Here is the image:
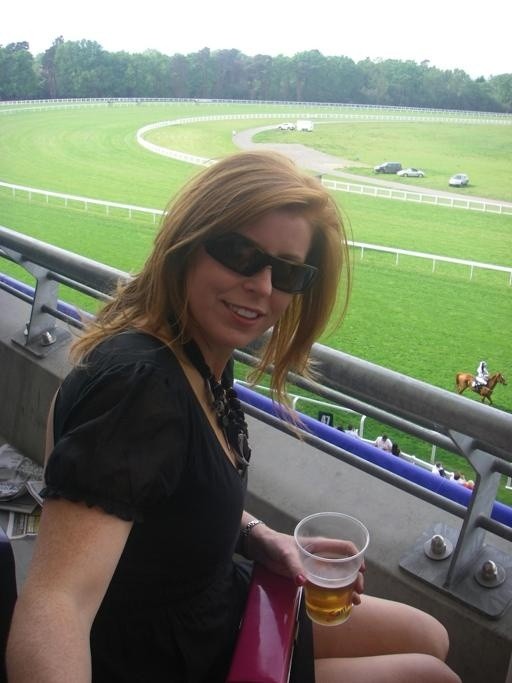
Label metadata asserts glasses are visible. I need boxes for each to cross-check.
[201,223,318,296]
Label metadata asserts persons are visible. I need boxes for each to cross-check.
[431,461,445,477]
[463,479,476,490]
[474,361,489,387]
[374,432,393,451]
[391,442,400,457]
[6,146,466,681]
[447,470,465,484]
[344,423,358,436]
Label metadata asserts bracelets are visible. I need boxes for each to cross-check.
[240,516,265,558]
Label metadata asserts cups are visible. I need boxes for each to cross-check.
[293,512,370,628]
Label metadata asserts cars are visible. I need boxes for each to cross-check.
[278,123,295,130]
[449,174,469,188]
[375,162,424,177]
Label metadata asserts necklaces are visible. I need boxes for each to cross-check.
[167,313,250,465]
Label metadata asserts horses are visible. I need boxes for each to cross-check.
[454,372,508,404]
